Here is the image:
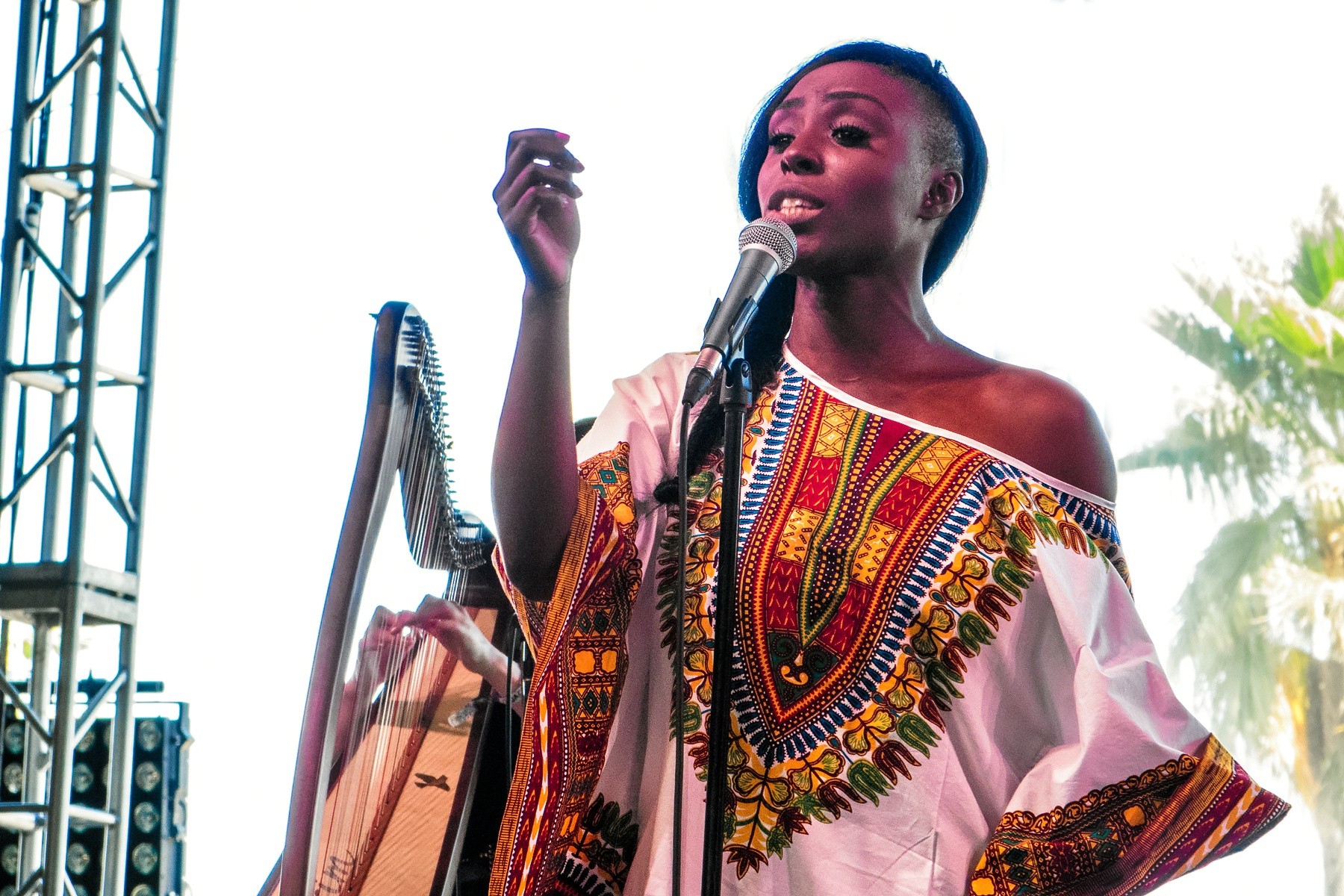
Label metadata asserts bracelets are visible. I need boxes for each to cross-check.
[447,682,523,729]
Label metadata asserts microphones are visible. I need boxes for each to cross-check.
[681,216,797,408]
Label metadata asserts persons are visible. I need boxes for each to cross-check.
[327,40,1292,895]
